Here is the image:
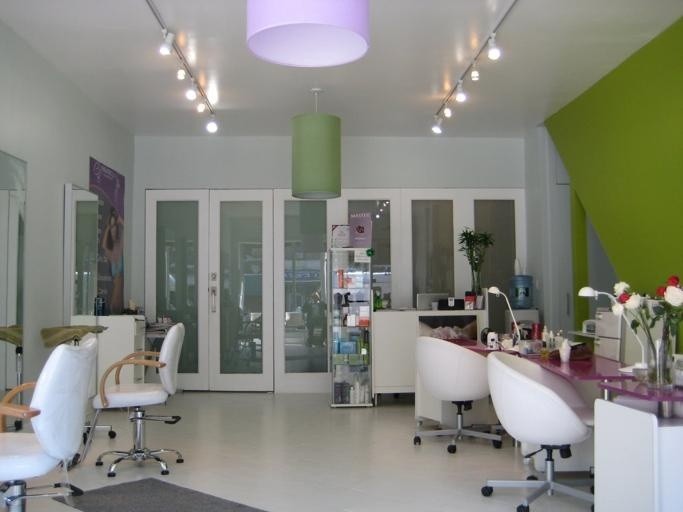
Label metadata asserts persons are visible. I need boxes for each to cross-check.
[102,205,125,315]
[300,289,329,348]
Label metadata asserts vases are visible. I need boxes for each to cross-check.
[649,338,674,390]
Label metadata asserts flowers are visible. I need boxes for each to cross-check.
[610,275,683,392]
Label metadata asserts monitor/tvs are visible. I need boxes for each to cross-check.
[416,293,449,310]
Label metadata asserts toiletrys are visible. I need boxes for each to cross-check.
[333,292,370,327]
[532,323,541,340]
[555,333,564,348]
[333,330,369,365]
[546,330,556,350]
[332,264,370,289]
[559,338,571,363]
[349,381,368,404]
[559,330,565,337]
[334,367,370,404]
[542,325,549,342]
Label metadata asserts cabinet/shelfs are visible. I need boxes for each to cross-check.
[97,315,146,382]
[373,288,491,410]
[326,249,376,412]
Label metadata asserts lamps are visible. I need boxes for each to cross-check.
[577,285,651,375]
[432,117,443,135]
[174,61,186,81]
[289,87,343,202]
[196,101,207,113]
[470,63,481,81]
[454,81,468,102]
[206,117,219,135]
[158,32,174,57]
[486,33,501,60]
[185,84,196,101]
[245,0,371,70]
[442,101,453,120]
[487,282,524,353]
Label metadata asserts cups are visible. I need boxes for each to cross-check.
[432,302,438,310]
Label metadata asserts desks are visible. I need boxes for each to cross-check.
[592,380,682,512]
[424,334,683,475]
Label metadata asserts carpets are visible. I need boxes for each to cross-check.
[54,478,267,512]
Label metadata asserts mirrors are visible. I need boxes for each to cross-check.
[65,182,101,331]
[0,151,28,435]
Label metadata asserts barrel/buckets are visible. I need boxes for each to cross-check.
[508,275,532,309]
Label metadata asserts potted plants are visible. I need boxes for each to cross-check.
[458,227,494,309]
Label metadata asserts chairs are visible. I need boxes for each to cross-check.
[412,333,505,457]
[480,349,592,512]
[1,331,96,512]
[91,322,188,475]
